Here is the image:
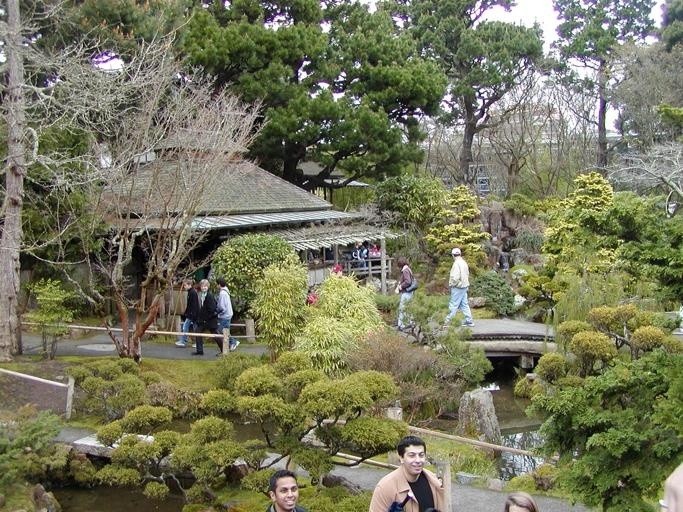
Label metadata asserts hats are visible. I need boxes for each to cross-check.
[452,248,461,255]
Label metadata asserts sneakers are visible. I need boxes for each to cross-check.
[230,341,240,351]
[191,343,196,347]
[462,322,474,326]
[192,351,203,355]
[215,351,222,356]
[175,341,185,347]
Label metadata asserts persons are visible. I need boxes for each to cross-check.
[393,255,417,329]
[350,241,379,281]
[191,278,223,356]
[174,276,200,349]
[503,492,538,512]
[333,259,341,274]
[306,289,314,305]
[308,251,314,263]
[214,279,240,354]
[368,435,450,512]
[441,246,475,327]
[264,469,307,512]
[658,461,682,512]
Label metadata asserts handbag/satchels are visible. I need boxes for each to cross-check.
[404,278,417,292]
